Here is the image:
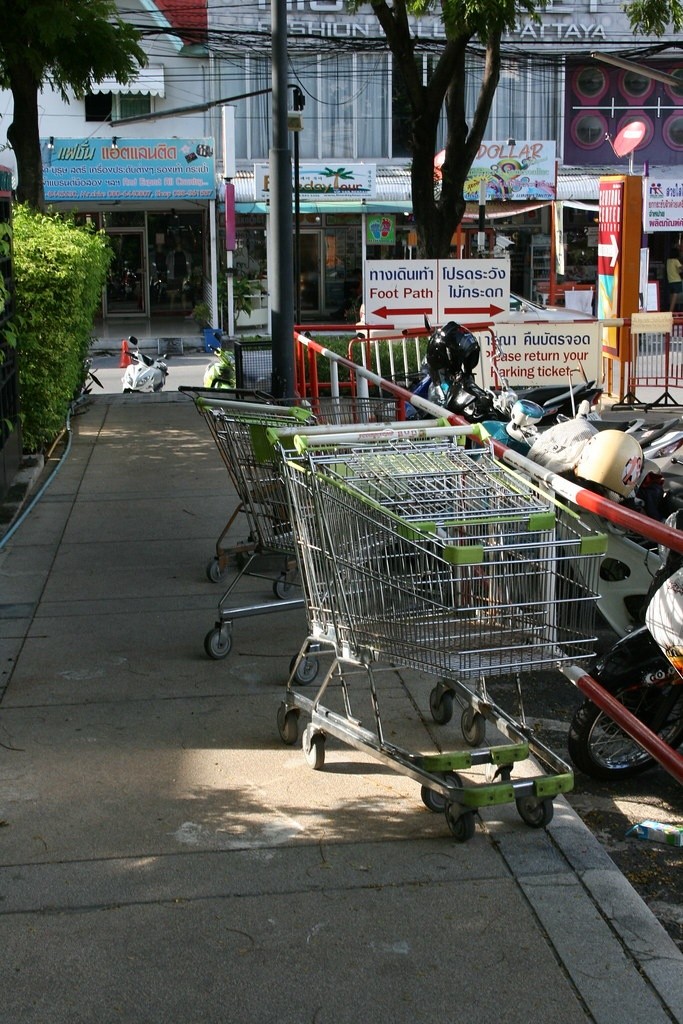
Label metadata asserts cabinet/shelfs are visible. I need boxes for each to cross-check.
[530,233,568,303]
[0,166,25,508]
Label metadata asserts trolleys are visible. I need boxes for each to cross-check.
[292,421,606,841]
[264,415,532,811]
[178,386,305,600]
[192,396,399,688]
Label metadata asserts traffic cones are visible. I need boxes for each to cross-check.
[118,340,134,368]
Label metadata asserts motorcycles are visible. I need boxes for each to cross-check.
[403,318,683,784]
[122,335,169,394]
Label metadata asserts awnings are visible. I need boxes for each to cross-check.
[72,62,165,99]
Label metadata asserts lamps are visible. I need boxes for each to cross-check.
[48,136,54,150]
[111,136,122,149]
[605,119,646,159]
[434,148,446,172]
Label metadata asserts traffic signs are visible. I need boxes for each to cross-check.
[596,181,624,276]
[362,259,438,331]
[437,258,511,325]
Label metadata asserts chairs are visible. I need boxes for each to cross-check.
[535,281,596,308]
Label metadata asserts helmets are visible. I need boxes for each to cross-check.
[576,430,644,498]
[426,319,479,373]
